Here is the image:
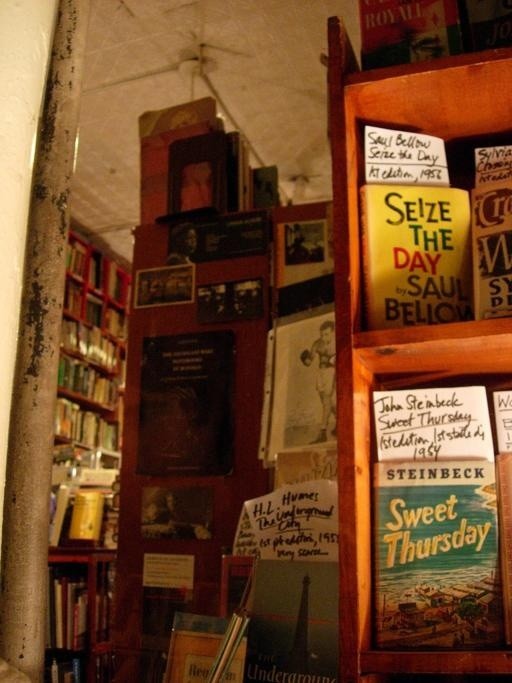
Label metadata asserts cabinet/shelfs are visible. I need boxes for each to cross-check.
[115,200,340,682]
[326,15,512,683]
[44,219,133,681]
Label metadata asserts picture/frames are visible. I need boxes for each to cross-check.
[134,264,196,308]
[195,276,268,324]
[247,558,339,682]
[258,303,337,466]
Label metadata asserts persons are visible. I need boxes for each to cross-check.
[162,221,200,266]
[298,321,338,443]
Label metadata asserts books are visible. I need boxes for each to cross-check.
[108,261,131,345]
[45,401,118,449]
[371,386,512,653]
[359,125,510,329]
[52,354,118,410]
[39,445,123,550]
[57,318,117,366]
[41,564,118,682]
[167,130,297,215]
[60,239,106,328]
